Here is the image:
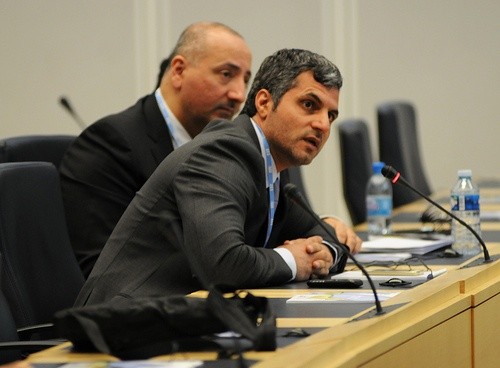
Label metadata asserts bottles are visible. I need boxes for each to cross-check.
[451,170,482,255]
[366,162,393,240]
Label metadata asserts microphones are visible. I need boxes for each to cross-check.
[60,98,86,130]
[381,166,490,261]
[284,183,382,313]
[160,212,247,368]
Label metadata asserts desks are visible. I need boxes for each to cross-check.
[0,186,500,368]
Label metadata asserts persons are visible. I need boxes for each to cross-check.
[60,21,363,283]
[72,48,349,310]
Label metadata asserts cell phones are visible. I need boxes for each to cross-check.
[307,280,363,289]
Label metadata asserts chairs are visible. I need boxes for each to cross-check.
[0,161,90,359]
[377,100,431,209]
[338,119,375,228]
